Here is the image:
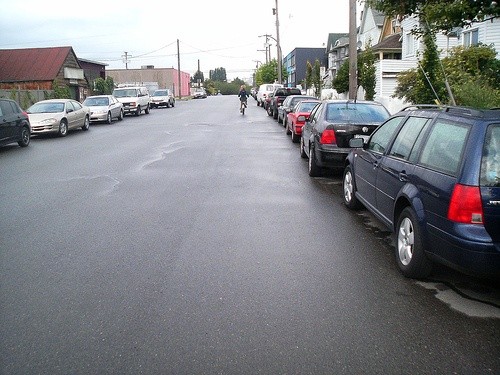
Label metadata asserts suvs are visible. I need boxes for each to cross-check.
[341,104,500,297]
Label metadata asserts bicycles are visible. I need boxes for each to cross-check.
[238,95,249,115]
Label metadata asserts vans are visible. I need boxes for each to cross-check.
[250,83,302,119]
[112,86,151,117]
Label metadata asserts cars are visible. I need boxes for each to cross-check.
[296,100,395,177]
[284,100,322,143]
[0,98,31,149]
[150,89,175,108]
[277,95,319,129]
[193,89,207,99]
[25,98,90,137]
[82,95,124,124]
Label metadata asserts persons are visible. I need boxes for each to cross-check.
[238,85,249,112]
